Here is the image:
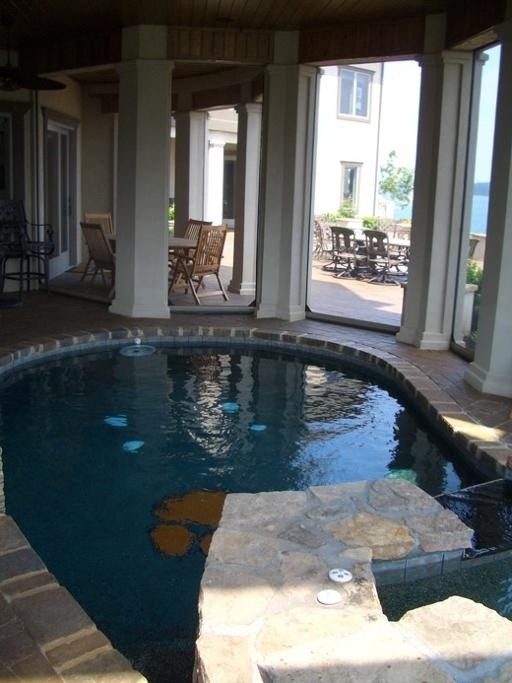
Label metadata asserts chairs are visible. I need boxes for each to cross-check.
[314,216,481,287]
[80,210,229,306]
[0,199,55,307]
